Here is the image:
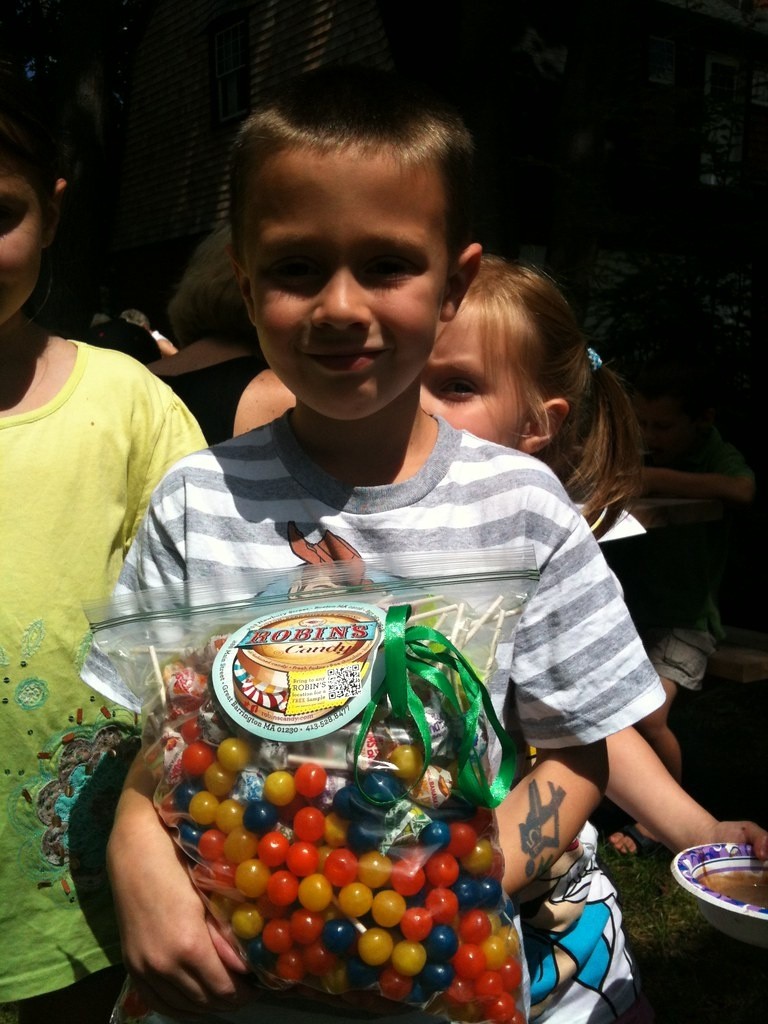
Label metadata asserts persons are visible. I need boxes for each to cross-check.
[77,83,768,1024]
[414,250,658,1022]
[0,70,209,1024]
[146,217,296,449]
[604,349,757,862]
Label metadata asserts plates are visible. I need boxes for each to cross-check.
[671,841,768,951]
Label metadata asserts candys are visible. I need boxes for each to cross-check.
[149,617,528,1024]
